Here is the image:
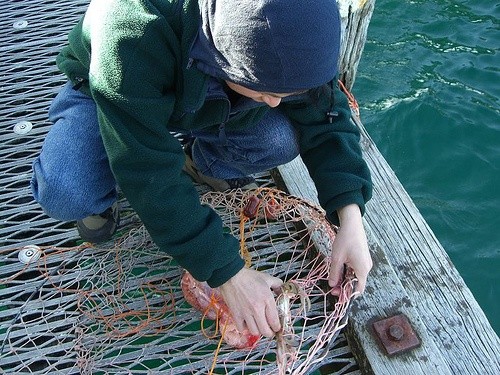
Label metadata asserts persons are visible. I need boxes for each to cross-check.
[31,0,374,338]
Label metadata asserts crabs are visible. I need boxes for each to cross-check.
[275,280,311,347]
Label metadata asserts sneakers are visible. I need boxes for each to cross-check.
[182,137,263,208]
[77,199,119,243]
